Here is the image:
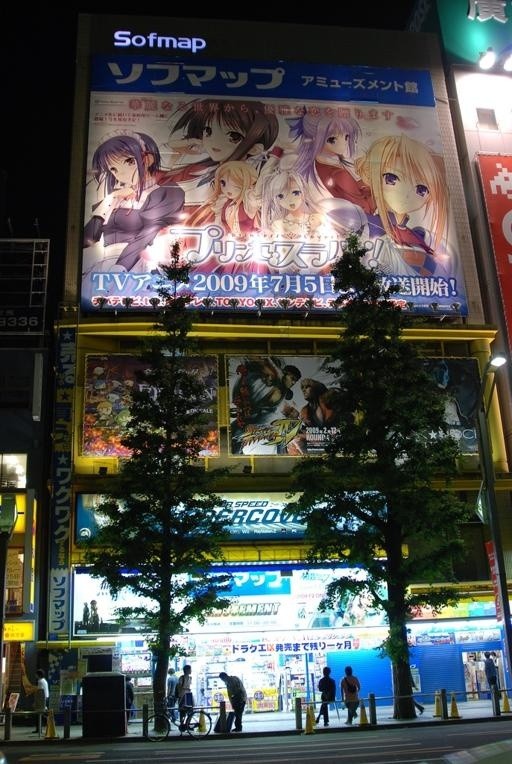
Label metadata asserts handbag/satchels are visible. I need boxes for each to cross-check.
[347,683,357,692]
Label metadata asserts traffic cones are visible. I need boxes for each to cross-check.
[357,697,369,725]
[194,700,209,733]
[431,688,445,718]
[43,706,61,740]
[498,686,512,713]
[307,699,318,727]
[448,691,462,717]
[303,706,316,735]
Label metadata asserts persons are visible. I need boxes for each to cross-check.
[316,667,335,727]
[126,676,133,724]
[167,664,194,725]
[31,669,50,734]
[484,651,501,698]
[341,667,360,725]
[228,354,356,457]
[219,672,247,732]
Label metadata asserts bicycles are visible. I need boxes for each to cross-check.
[141,686,213,742]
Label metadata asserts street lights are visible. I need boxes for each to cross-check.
[467,349,511,691]
